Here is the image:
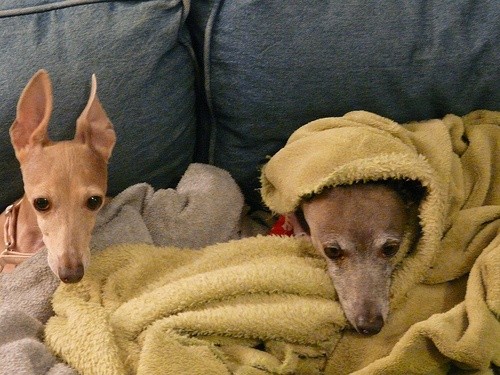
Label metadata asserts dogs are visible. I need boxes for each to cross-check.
[299,184,426,334]
[1,69,116,285]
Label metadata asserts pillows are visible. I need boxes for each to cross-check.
[1,0,202,212]
[187,0,500,213]
